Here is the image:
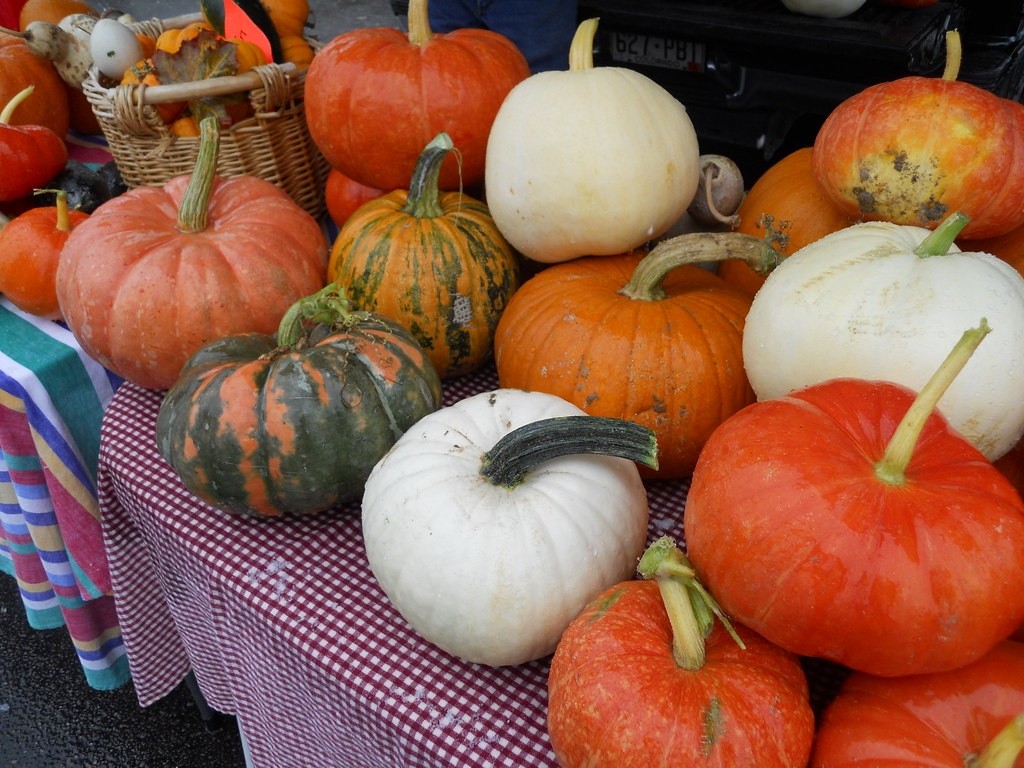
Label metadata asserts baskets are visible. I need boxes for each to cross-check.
[80,9,331,225]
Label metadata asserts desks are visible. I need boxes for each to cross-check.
[0,131,694,768]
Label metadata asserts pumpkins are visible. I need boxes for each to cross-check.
[0,0,1024,768]
